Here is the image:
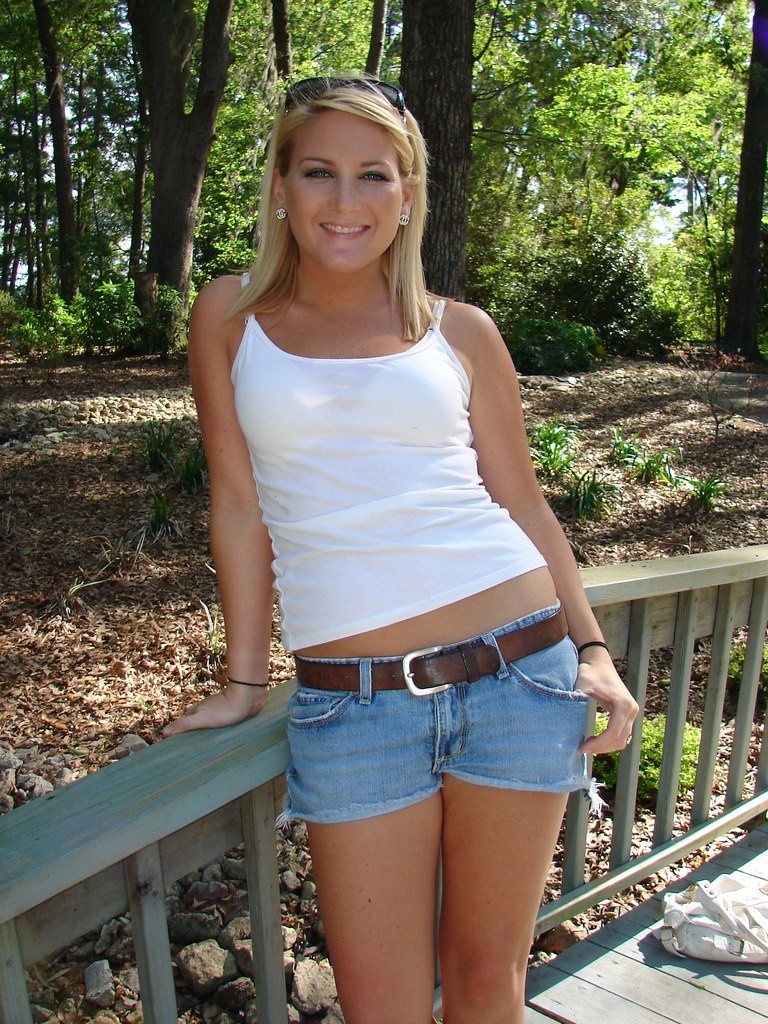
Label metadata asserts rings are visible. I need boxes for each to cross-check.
[627,736,632,744]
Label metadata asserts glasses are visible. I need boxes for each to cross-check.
[283,77,411,142]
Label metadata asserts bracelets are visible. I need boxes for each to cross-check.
[228,677,269,687]
[578,640,609,654]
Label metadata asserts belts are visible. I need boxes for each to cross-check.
[294,602,568,696]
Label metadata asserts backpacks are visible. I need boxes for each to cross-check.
[652,874,768,963]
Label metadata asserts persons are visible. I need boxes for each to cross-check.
[163,76,642,1024]
